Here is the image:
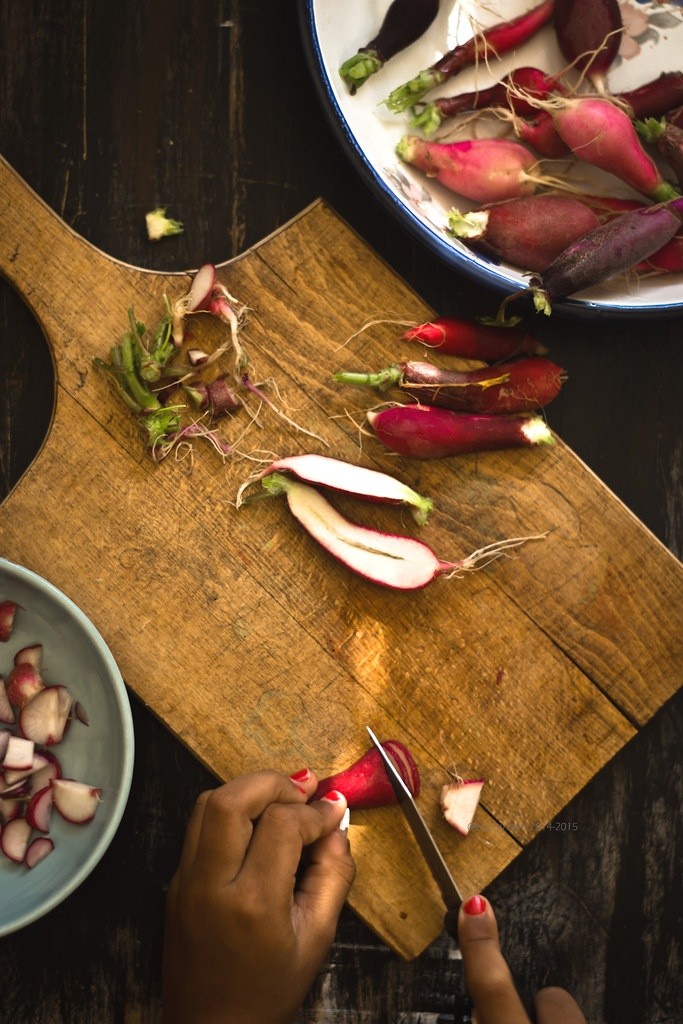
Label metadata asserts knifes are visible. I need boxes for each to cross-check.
[367,726,463,943]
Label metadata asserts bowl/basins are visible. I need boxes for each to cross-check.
[0,559,134,936]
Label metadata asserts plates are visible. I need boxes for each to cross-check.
[312,0,682,313]
[0,265,58,506]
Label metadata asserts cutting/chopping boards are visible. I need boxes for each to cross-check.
[0,158,682,957]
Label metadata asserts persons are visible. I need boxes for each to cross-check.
[159,768,588,1024]
